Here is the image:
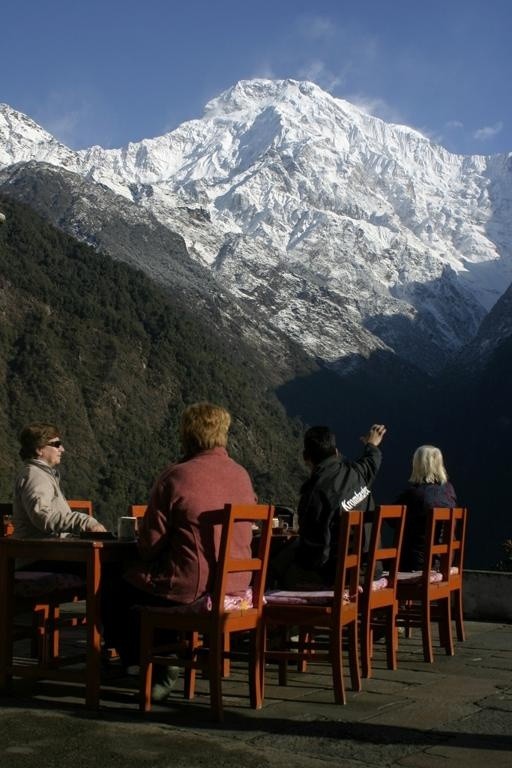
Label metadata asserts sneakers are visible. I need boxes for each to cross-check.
[134,665,178,701]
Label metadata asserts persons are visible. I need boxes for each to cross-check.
[99,402,258,699]
[265,422,386,651]
[374,443,459,640]
[12,425,106,656]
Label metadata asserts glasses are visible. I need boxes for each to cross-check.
[47,439,63,448]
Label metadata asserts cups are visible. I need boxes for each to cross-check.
[111,517,136,538]
[272,518,289,534]
